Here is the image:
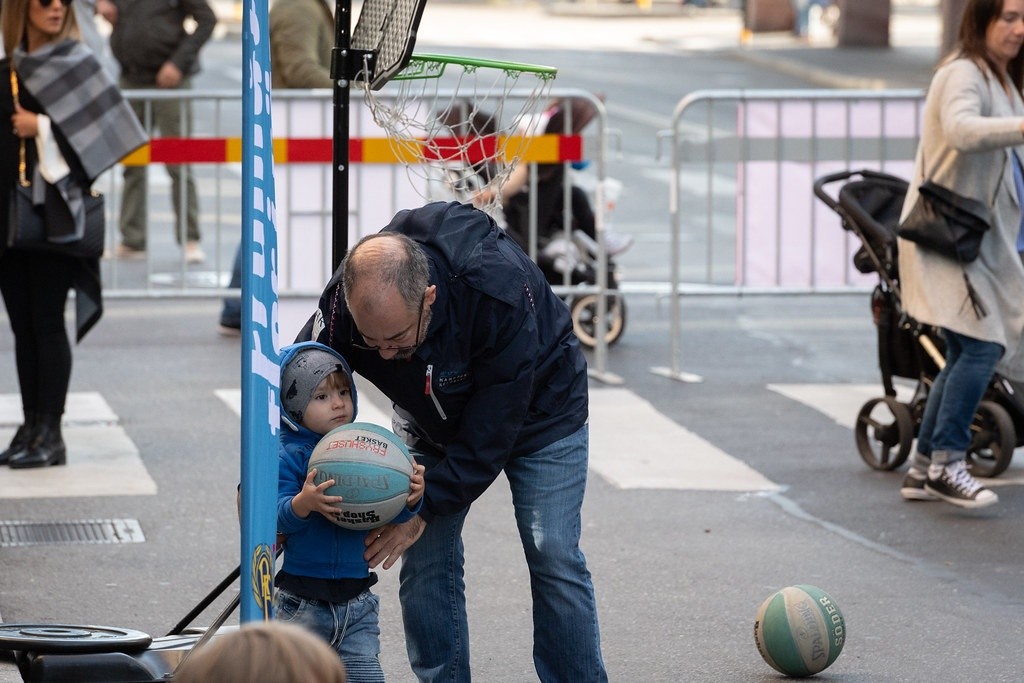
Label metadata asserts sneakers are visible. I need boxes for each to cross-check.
[924,460,999,508]
[901,472,941,501]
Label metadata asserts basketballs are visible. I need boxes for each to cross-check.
[307,422,416,531]
[755,585,846,675]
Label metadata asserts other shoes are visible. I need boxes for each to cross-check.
[102,244,147,261]
[184,240,203,262]
[8,434,66,468]
[0,426,40,465]
[219,302,241,337]
[604,235,632,258]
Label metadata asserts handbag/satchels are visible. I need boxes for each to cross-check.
[897,179,992,321]
[7,183,106,257]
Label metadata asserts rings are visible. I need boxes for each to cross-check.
[13,129,18,134]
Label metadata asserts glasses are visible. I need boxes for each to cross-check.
[39,0,72,8]
[350,287,426,352]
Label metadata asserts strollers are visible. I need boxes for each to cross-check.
[442,90,625,347]
[812,170,1024,479]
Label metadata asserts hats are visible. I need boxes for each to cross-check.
[280,348,350,427]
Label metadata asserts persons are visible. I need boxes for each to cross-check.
[276,339,426,683]
[480,109,599,283]
[98,0,218,264]
[235,198,612,683]
[897,0,1024,510]
[0,0,154,470]
[218,0,369,337]
[173,620,347,683]
